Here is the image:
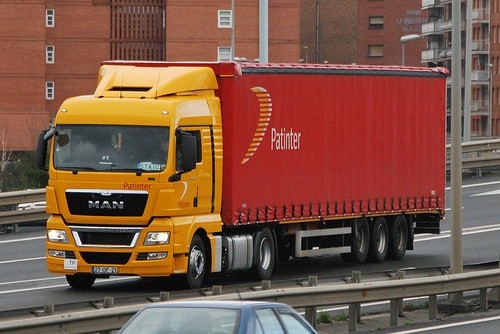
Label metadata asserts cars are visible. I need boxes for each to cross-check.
[117,301,320,334]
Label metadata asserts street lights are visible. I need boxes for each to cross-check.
[398,33,419,67]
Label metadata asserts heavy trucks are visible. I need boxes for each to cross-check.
[36,59,450,290]
